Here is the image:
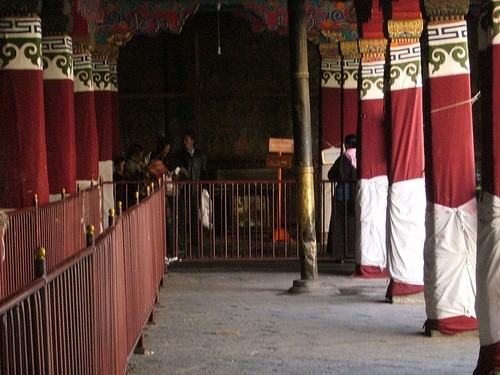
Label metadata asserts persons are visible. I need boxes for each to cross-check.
[114,137,180,258]
[172,131,210,258]
[327,134,357,262]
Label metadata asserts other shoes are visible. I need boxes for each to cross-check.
[189,237,198,247]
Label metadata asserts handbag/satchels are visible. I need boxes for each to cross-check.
[198,188,213,230]
[163,169,183,197]
